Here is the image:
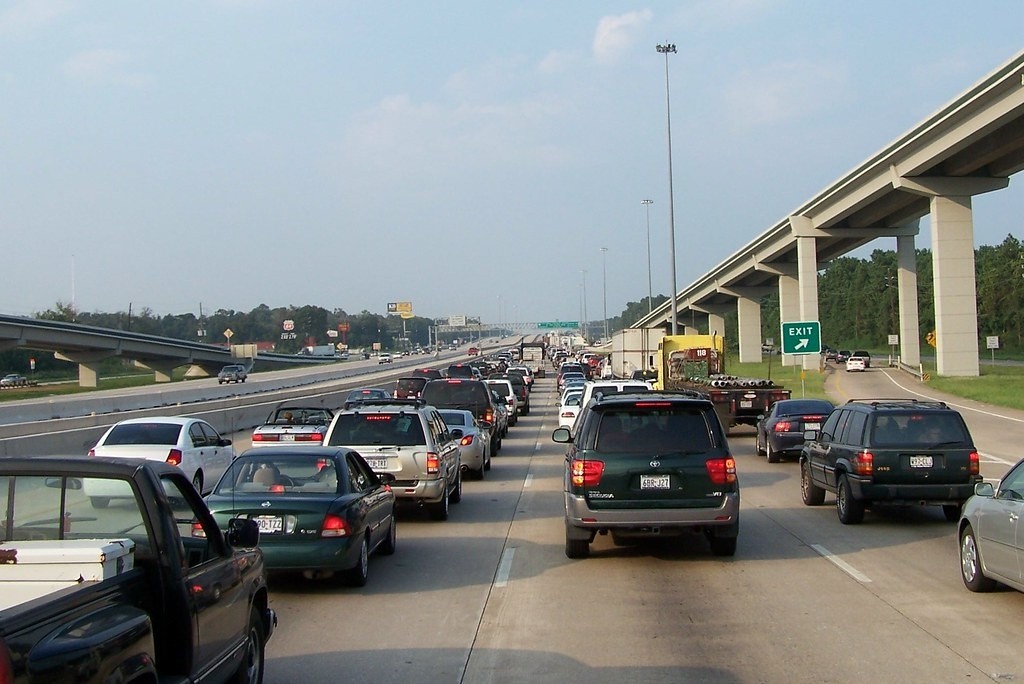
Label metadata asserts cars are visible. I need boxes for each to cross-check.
[0,374,28,388]
[251,331,645,455]
[435,406,495,479]
[83,417,238,510]
[761,343,774,352]
[191,444,398,588]
[821,345,871,372]
[755,399,836,465]
[954,457,1024,595]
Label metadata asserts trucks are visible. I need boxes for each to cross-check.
[632,330,792,437]
[611,327,667,383]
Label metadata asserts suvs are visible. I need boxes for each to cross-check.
[323,397,466,520]
[553,389,740,561]
[217,365,247,385]
[801,397,985,524]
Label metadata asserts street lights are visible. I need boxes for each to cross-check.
[655,40,678,336]
[600,248,609,343]
[580,269,589,339]
[641,199,653,314]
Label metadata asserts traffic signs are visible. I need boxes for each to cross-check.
[782,321,822,356]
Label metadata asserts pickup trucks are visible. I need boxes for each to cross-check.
[0,456,278,684]
